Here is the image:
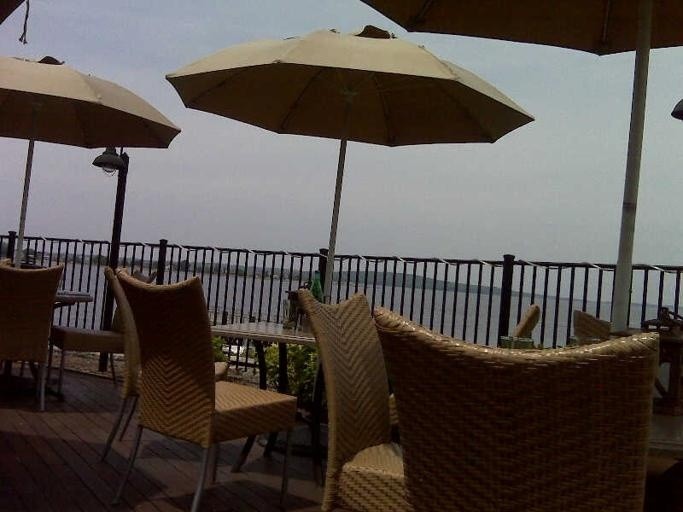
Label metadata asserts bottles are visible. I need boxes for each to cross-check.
[310,270,323,304]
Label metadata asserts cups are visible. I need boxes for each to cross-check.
[295,313,312,337]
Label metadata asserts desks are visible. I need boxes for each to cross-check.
[210,321,327,496]
[19,291,94,395]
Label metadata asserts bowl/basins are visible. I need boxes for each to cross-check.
[500,336,534,349]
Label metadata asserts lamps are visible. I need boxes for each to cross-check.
[90,140,127,176]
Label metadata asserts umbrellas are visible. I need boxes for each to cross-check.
[0,55,181,269]
[360,1,683,341]
[165,24,536,450]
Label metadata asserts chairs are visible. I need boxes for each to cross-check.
[49,272,159,402]
[2,264,62,411]
[374,307,659,512]
[301,289,408,512]
[385,303,683,453]
[100,266,229,459]
[113,271,297,512]
